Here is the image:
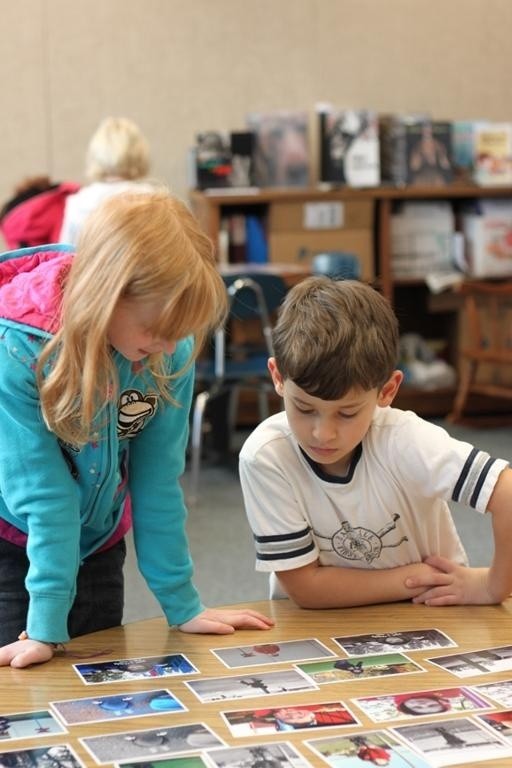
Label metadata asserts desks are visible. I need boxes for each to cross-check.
[0,597,512,768]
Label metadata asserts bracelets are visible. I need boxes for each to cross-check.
[17,630,57,649]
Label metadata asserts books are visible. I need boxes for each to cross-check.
[194,108,512,189]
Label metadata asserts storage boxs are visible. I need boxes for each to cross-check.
[460,199,512,281]
[388,202,455,275]
[262,199,376,285]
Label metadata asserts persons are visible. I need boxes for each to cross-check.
[251,704,324,725]
[237,273,512,610]
[0,188,276,669]
[0,173,82,250]
[334,659,364,674]
[351,736,391,766]
[359,688,463,716]
[435,726,466,751]
[58,116,162,249]
[94,657,170,673]
[346,630,436,645]
[240,677,268,689]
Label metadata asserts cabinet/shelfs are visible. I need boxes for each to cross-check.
[188,183,512,431]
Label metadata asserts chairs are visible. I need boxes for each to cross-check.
[443,278,512,426]
[185,271,289,512]
